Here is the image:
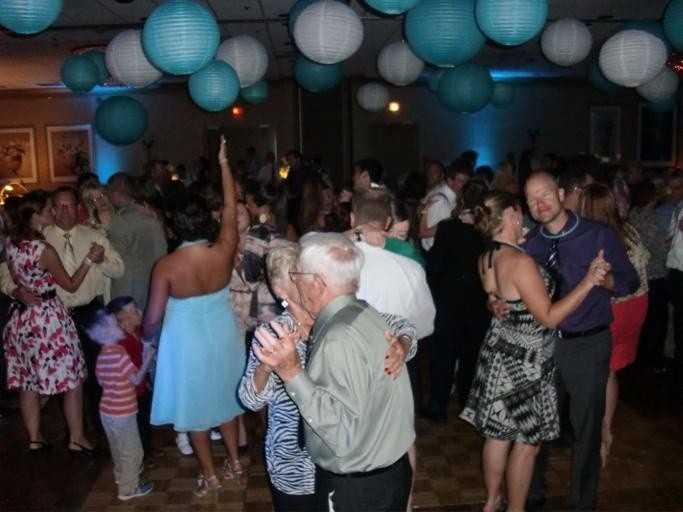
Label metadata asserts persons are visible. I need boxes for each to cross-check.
[1,143,683,512]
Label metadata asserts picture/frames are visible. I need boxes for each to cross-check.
[586,103,623,165]
[44,122,95,184]
[0,126,39,186]
[636,99,678,170]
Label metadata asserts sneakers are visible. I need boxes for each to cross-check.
[114,463,145,485]
[646,364,672,377]
[117,479,154,502]
[209,430,223,441]
[173,433,193,457]
[428,400,448,424]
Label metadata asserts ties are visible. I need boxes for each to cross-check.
[297,324,314,452]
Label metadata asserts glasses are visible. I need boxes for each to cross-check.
[286,269,316,281]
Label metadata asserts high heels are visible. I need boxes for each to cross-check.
[28,440,54,458]
[222,458,247,480]
[192,474,223,500]
[65,439,101,461]
[599,431,614,469]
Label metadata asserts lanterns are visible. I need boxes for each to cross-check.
[59,2,269,143]
[587,1,683,115]
[541,20,592,66]
[288,0,548,116]
[0,0,65,34]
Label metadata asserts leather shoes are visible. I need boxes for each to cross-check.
[238,443,249,456]
